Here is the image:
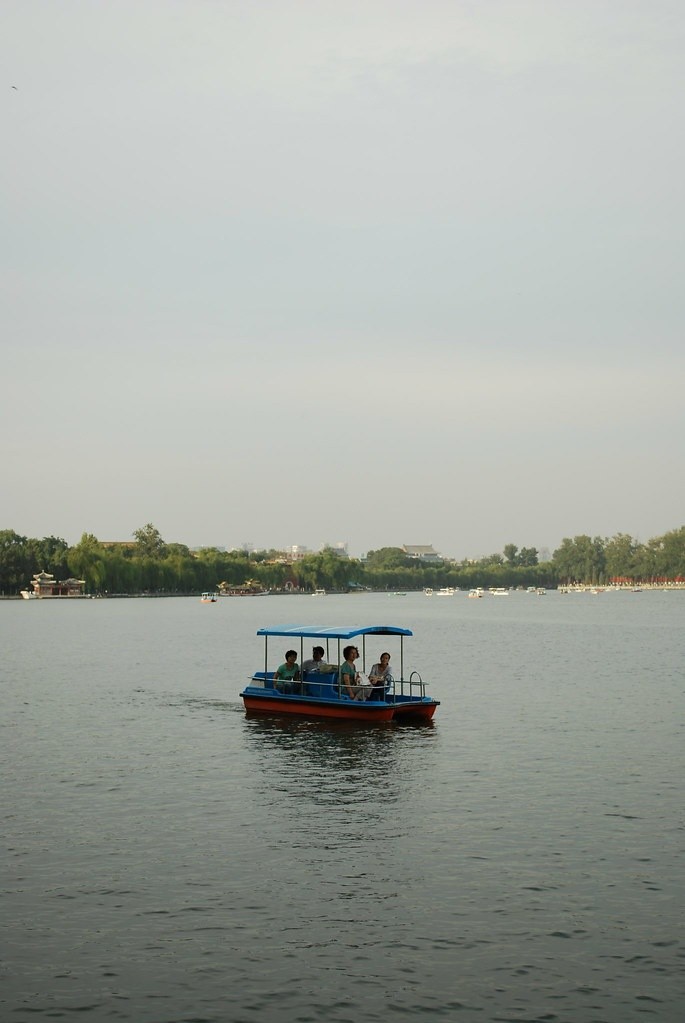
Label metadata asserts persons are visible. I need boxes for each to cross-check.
[300,646,326,672]
[368,652,392,701]
[273,650,300,694]
[341,646,373,702]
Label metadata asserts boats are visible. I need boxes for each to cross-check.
[216,578,271,596]
[312,589,327,597]
[421,585,550,598]
[238,623,441,723]
[199,590,219,603]
